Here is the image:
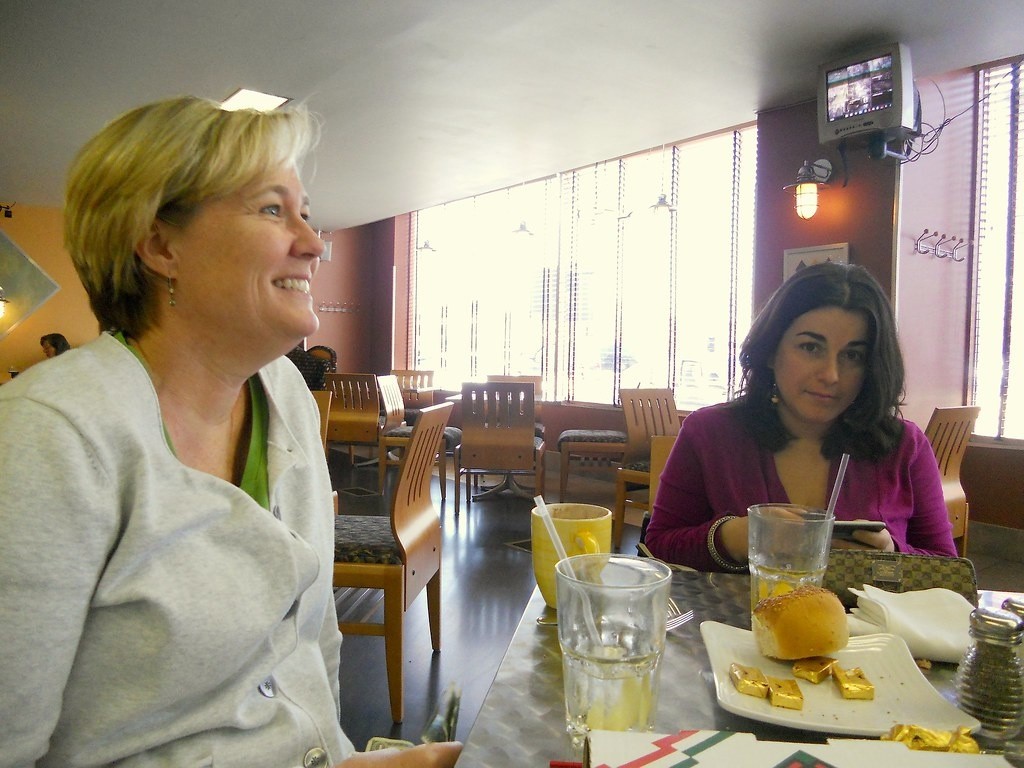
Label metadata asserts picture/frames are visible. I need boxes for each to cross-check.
[782,242,851,284]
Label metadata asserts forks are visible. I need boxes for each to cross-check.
[536,609,693,632]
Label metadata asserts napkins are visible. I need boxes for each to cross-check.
[840,582,985,663]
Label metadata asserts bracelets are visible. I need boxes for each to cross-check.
[706,514,748,573]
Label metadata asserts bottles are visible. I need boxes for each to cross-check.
[954,598,1024,741]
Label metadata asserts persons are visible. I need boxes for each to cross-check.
[644,263,959,577]
[40,333,70,358]
[0,97,465,768]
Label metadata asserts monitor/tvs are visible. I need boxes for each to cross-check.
[817,43,921,152]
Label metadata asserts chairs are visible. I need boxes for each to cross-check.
[279,365,981,724]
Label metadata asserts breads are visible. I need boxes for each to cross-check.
[752,584,850,659]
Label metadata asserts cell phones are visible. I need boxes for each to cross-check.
[831,522,886,547]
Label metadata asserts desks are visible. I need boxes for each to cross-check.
[448,553,1024,767]
[445,391,561,501]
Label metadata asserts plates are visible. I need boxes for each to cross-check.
[700,619,981,734]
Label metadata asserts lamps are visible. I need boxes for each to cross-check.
[0,286,11,317]
[782,158,836,221]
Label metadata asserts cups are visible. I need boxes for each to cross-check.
[555,552,672,743]
[531,502,612,610]
[747,502,836,631]
